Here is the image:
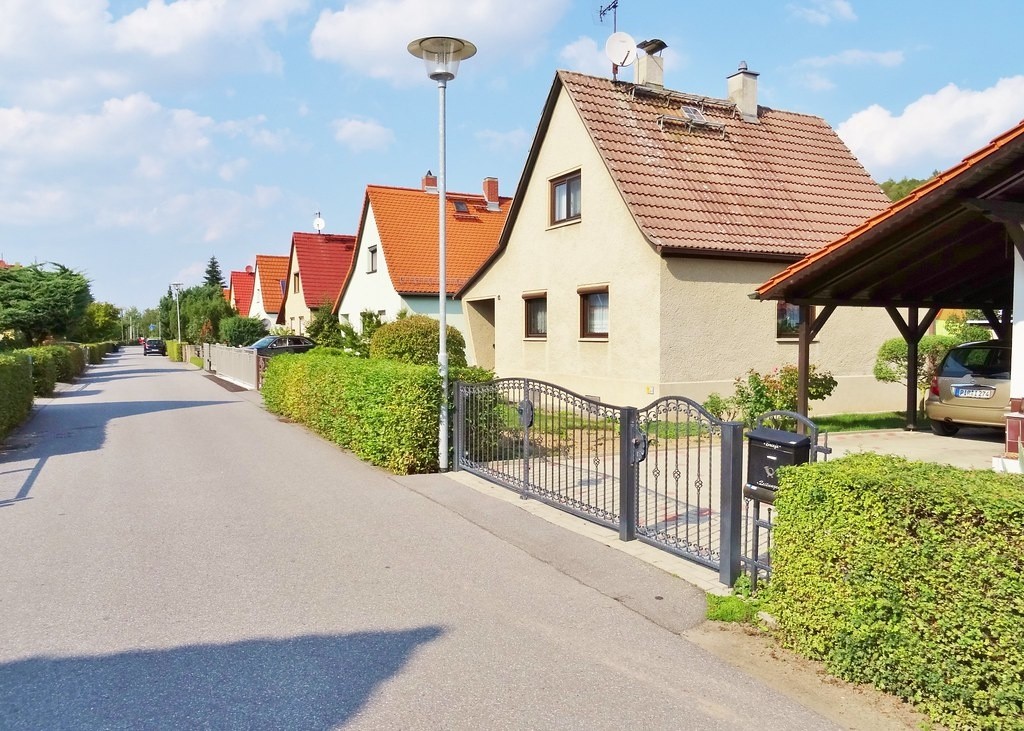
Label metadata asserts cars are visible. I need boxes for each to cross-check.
[244,334,318,358]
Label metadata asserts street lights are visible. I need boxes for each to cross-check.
[170,281,184,343]
[404,34,479,477]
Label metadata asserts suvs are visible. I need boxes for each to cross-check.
[142,339,166,356]
[926,338,1012,436]
